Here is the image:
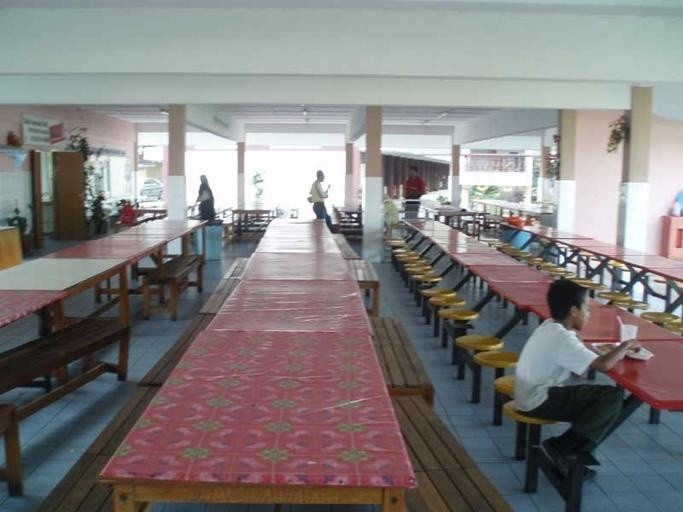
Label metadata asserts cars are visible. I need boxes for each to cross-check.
[136,178,165,202]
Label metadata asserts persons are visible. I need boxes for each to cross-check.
[404,166,426,219]
[193,175,215,221]
[512,277,641,482]
[306,170,334,232]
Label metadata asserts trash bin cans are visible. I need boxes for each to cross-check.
[500,222,531,253]
[197,220,225,260]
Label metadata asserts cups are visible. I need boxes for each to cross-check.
[620,325,637,343]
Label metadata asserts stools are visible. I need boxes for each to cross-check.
[501,399,559,492]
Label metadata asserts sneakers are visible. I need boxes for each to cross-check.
[540,438,561,470]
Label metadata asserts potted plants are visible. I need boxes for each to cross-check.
[85,194,108,235]
[4,197,41,255]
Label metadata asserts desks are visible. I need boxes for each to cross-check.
[1,240,130,381]
